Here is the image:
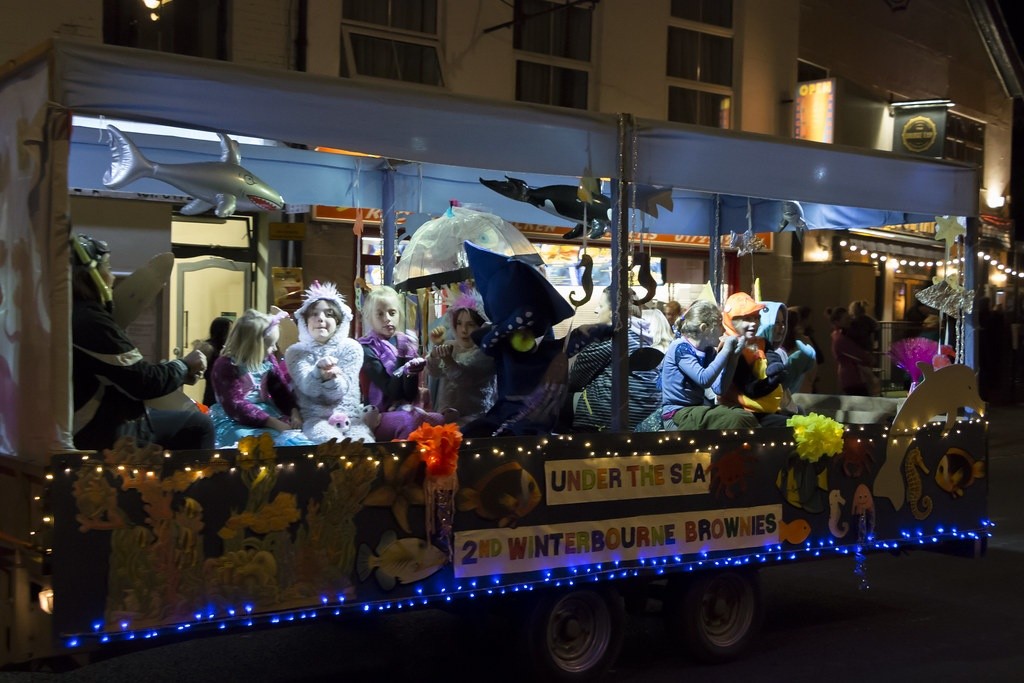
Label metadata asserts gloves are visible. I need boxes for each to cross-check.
[729,334,745,367]
[719,336,738,360]
[785,340,816,380]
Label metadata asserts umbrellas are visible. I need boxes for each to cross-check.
[395,206,543,293]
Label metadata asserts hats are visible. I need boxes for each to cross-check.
[725,292,765,319]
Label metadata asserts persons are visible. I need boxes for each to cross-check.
[285,282,365,443]
[71,235,880,448]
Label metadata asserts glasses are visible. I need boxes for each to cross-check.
[97,256,112,269]
